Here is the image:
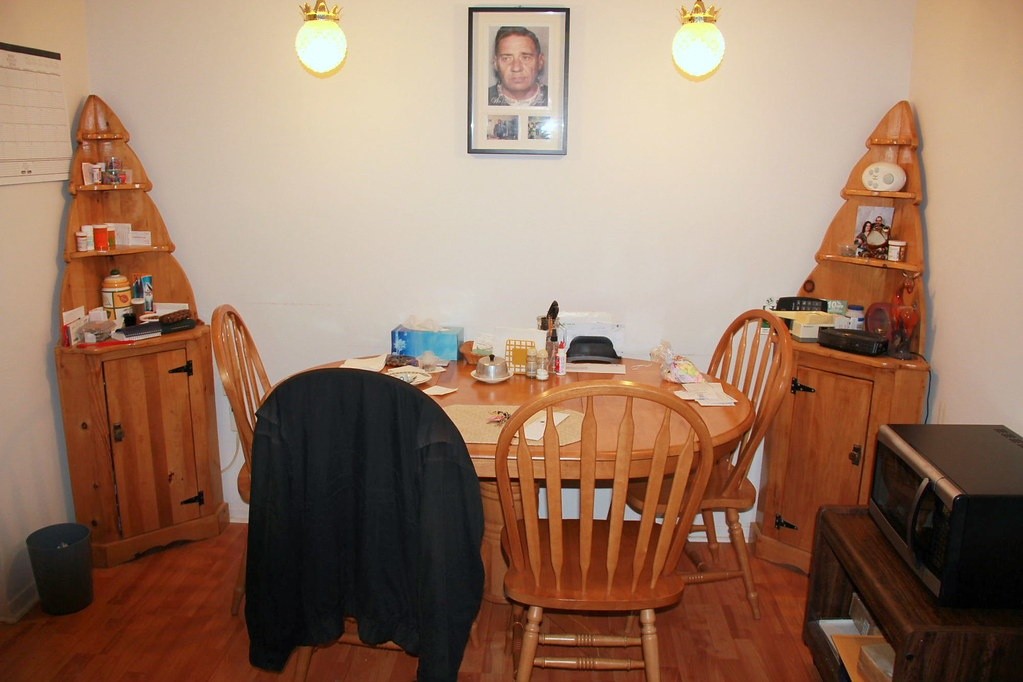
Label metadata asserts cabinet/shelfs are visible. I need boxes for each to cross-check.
[55,95,229,568]
[748,99,931,575]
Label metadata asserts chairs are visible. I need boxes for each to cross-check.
[252,366,483,682]
[607,310,795,619]
[211,305,273,617]
[478,379,713,682]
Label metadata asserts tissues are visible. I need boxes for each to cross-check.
[390,313,464,361]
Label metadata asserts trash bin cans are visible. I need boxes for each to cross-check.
[26,522,94,615]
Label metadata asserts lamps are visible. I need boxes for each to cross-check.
[672,0,726,76]
[294,0,346,74]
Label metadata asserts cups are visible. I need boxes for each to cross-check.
[887,240,908,262]
[130,299,146,322]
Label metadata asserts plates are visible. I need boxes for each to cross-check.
[471,369,514,383]
[385,366,432,386]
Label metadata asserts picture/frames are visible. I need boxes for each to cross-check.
[466,6,570,155]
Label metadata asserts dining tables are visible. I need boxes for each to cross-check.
[257,357,754,606]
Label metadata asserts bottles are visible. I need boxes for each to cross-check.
[526,348,538,379]
[536,349,550,381]
[74,224,116,252]
[845,305,864,330]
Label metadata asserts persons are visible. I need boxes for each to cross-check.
[494,120,505,140]
[488,26,549,107]
[872,216,886,233]
[854,221,871,249]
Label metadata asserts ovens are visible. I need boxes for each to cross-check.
[867,423,1023,605]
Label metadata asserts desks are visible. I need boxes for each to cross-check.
[801,505,1023,682]
